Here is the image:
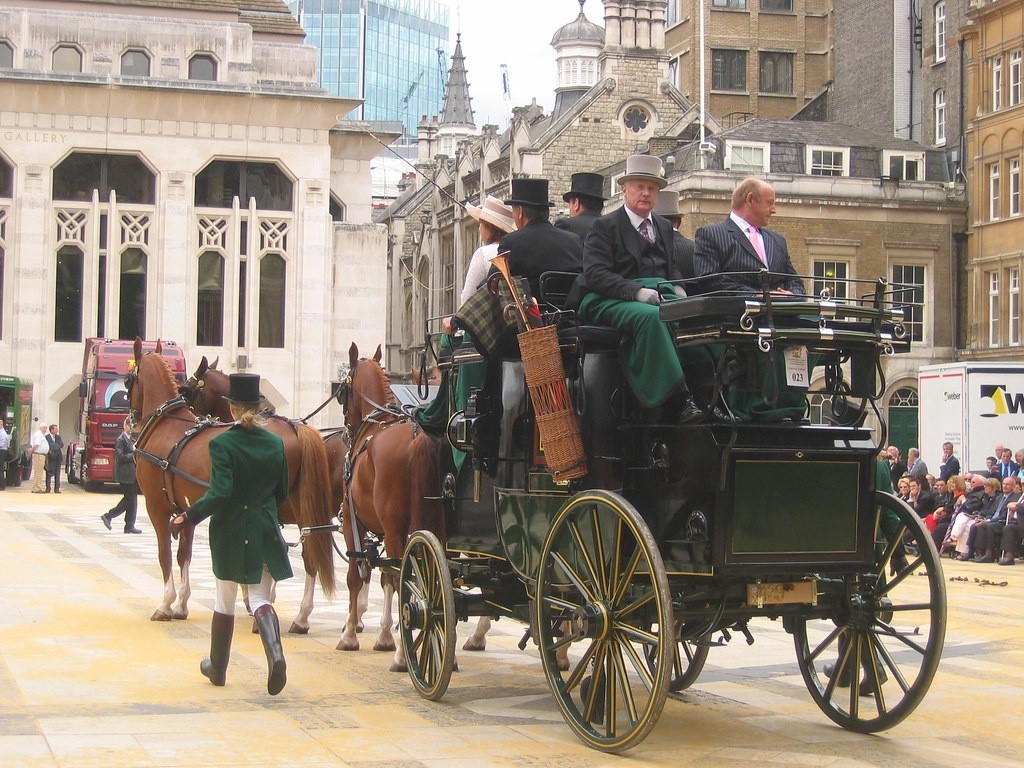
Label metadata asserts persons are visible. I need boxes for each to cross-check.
[877,443,1024,566]
[556,169,610,244]
[461,196,518,308]
[652,190,695,280]
[442,179,585,336]
[45,424,64,494]
[696,179,811,425]
[28,424,49,493]
[100,416,143,534]
[171,373,294,696]
[823,457,913,697]
[567,155,742,424]
[0,420,21,491]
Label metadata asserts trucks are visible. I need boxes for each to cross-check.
[0,375,33,488]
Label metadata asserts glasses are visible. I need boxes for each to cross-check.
[965,478,971,481]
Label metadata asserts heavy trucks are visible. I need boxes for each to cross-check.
[66,338,187,492]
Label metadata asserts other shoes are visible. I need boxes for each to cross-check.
[53,490,61,493]
[45,489,50,493]
[31,489,45,494]
[124,525,143,534]
[791,417,812,426]
[904,545,1016,565]
[100,515,112,530]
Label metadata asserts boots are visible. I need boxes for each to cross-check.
[254,604,287,696]
[855,639,889,698]
[199,611,235,687]
[822,632,853,688]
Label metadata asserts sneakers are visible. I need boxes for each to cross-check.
[704,402,741,425]
[680,398,703,424]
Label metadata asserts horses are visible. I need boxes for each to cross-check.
[129,334,574,673]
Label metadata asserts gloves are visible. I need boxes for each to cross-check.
[636,288,661,306]
[889,556,914,578]
[672,284,688,298]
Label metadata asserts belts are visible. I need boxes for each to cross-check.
[33,452,47,456]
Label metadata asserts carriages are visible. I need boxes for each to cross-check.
[123,268,949,753]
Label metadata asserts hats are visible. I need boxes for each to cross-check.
[651,191,686,219]
[221,373,268,404]
[504,177,557,207]
[464,194,518,233]
[618,154,668,188]
[563,172,609,203]
[898,478,910,488]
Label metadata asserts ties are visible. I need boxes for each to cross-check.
[639,220,649,242]
[748,226,763,264]
[1002,464,1009,479]
[995,496,1006,513]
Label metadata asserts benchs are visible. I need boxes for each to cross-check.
[542,270,835,354]
